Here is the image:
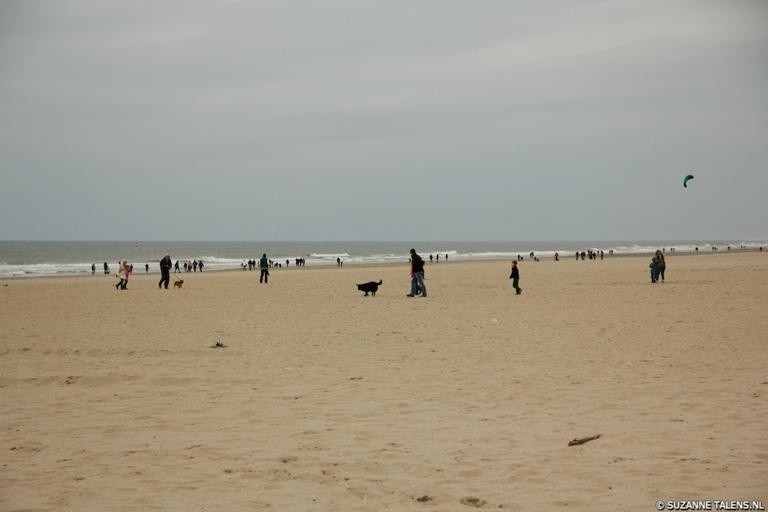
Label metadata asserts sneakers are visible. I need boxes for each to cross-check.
[406,289,427,298]
[114,283,170,290]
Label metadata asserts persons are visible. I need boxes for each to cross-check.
[90,247,666,299]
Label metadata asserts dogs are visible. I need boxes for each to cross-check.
[173,280,184,288]
[356,279,383,296]
